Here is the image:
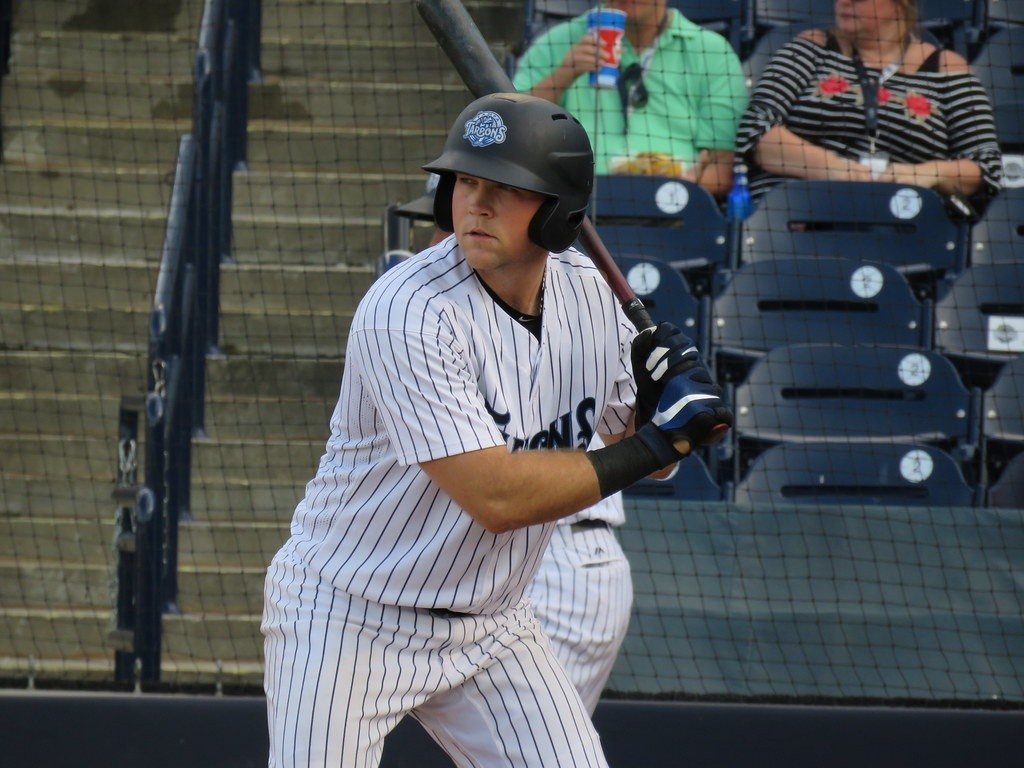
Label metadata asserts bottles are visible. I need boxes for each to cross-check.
[728,166,752,219]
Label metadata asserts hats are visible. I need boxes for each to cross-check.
[394,173,440,221]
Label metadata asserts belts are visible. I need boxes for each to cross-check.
[429,608,468,619]
[571,518,607,528]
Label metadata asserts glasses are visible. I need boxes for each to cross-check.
[621,63,649,108]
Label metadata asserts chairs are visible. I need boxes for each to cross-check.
[381,0,1024,508]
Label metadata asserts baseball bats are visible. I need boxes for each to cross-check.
[415,0,729,448]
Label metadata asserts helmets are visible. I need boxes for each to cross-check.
[422,93,594,254]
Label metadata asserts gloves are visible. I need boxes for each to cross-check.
[633,366,734,469]
[631,322,705,414]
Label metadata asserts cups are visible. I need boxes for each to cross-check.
[587,8,626,87]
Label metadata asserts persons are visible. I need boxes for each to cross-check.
[735,0,1004,316]
[260,93,733,768]
[511,1,748,195]
